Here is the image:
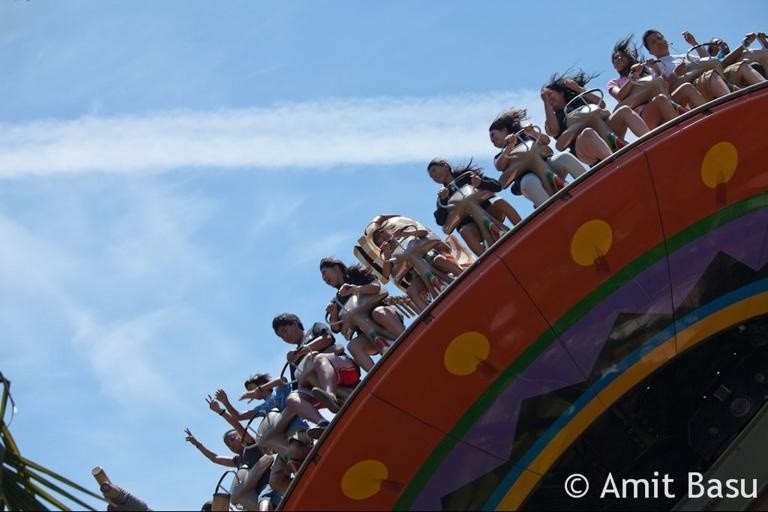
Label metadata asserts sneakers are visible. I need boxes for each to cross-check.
[307,421,329,439]
[312,387,340,413]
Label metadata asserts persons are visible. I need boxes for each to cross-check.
[366,212,464,311]
[184,313,361,512]
[603,26,768,133]
[318,256,409,372]
[488,109,589,210]
[428,154,521,258]
[539,66,651,168]
